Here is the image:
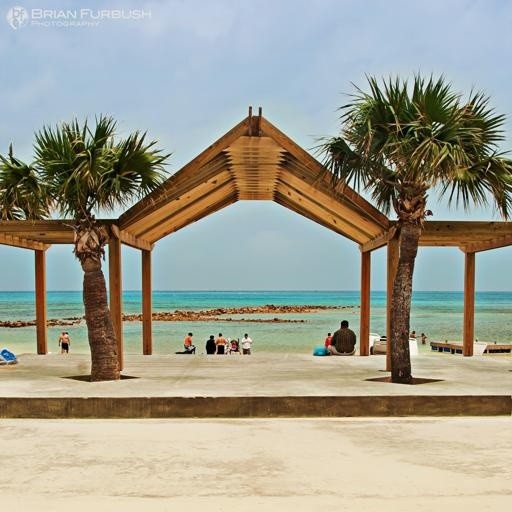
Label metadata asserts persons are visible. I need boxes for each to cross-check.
[410,330,417,338]
[325,331,332,347]
[183,330,253,354]
[420,333,428,345]
[59,331,72,354]
[329,320,357,355]
